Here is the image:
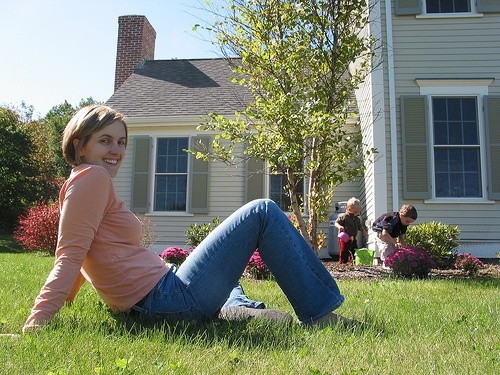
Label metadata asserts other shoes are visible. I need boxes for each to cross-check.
[218,307,293,324]
[299,312,384,335]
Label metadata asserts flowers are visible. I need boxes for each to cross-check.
[453,253,486,273]
[160,246,190,269]
[384,246,437,272]
[245,247,270,278]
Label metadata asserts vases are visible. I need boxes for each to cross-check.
[256,272,271,280]
[393,267,432,281]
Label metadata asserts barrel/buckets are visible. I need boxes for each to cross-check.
[354,249,375,266]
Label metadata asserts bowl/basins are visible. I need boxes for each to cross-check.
[338,231,351,242]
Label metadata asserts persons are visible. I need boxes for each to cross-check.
[371,205,418,270]
[0,104,368,342]
[334,196,368,265]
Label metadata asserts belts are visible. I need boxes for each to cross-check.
[130,298,144,320]
